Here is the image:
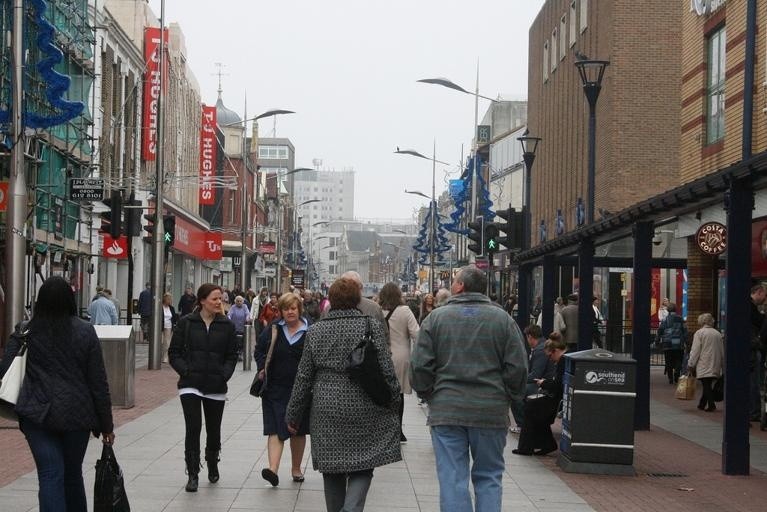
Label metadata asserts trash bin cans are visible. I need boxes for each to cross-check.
[86,324,136,410]
[556,348,638,476]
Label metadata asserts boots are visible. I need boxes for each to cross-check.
[185,450,203,492]
[205,443,221,483]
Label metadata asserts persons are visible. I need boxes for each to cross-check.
[654,303,687,385]
[253,292,315,487]
[504,293,604,456]
[408,266,529,512]
[0,275,116,512]
[167,283,239,492]
[85,286,121,326]
[657,297,669,327]
[748,281,767,427]
[687,313,724,412]
[377,282,421,443]
[284,277,403,511]
[137,270,452,371]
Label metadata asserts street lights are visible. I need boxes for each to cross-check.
[416,66,500,262]
[263,165,332,287]
[575,61,614,349]
[515,131,544,331]
[240,92,295,298]
[386,137,450,295]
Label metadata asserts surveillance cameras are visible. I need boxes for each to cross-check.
[652,234,662,245]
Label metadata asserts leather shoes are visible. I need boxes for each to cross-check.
[292,468,304,482]
[262,469,279,486]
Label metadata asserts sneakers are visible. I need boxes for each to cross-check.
[512,449,532,456]
[535,445,557,455]
[510,426,521,433]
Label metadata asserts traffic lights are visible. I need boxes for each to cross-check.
[143,213,156,246]
[100,193,119,240]
[495,205,516,250]
[164,216,175,245]
[467,216,483,257]
[486,220,500,255]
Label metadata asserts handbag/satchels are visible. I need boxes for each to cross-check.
[250,373,263,397]
[0,342,29,421]
[94,443,131,511]
[713,376,723,402]
[349,317,392,406]
[675,370,698,400]
